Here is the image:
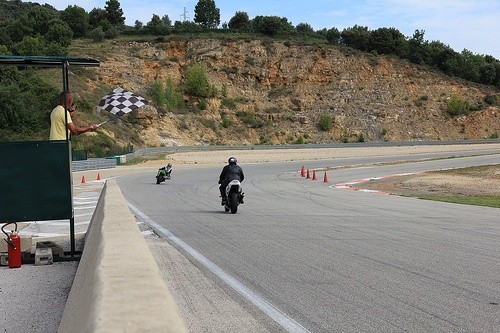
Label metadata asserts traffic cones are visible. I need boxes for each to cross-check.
[323,172,328,183]
[301,166,306,177]
[81,175,85,183]
[312,170,317,180]
[97,173,100,180]
[306,169,310,178]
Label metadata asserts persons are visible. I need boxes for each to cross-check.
[218,158,244,205]
[156,164,172,178]
[49,92,98,140]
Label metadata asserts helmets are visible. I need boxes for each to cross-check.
[228,157,237,163]
[168,163,172,167]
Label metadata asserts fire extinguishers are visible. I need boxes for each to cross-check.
[2,221,21,268]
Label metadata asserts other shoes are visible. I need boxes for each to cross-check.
[240,195,244,204]
[221,195,226,206]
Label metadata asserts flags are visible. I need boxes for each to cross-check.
[97,87,149,117]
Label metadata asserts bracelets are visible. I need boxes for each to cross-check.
[91,126,93,131]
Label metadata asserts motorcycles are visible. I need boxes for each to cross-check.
[156,169,170,184]
[218,180,245,214]
